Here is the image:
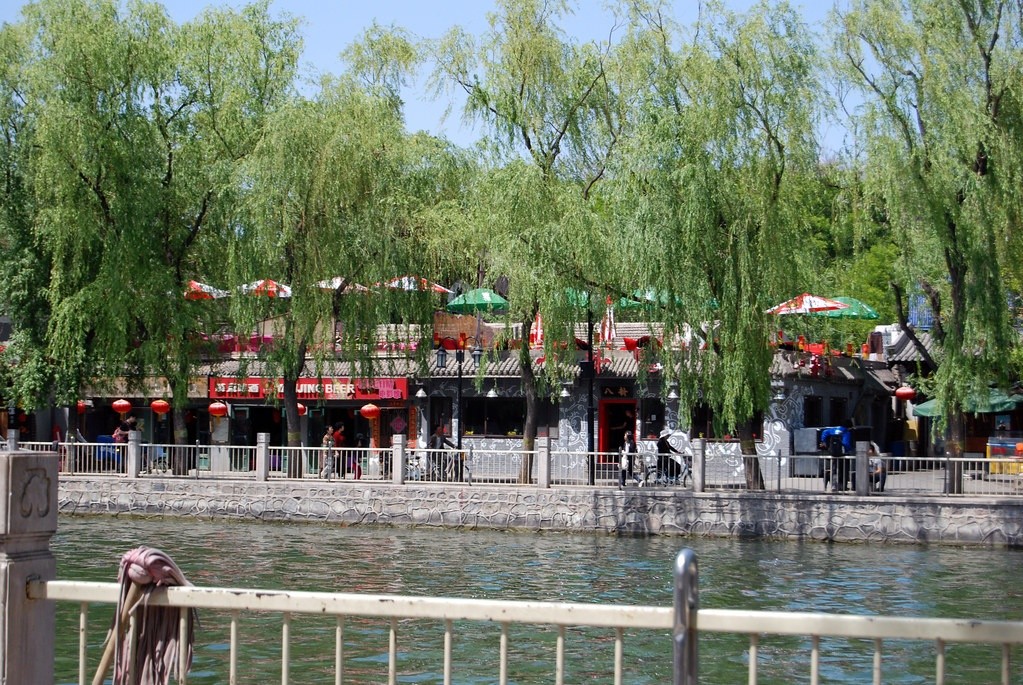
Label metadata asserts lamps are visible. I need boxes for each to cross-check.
[559,388,571,397]
[414,387,428,398]
[485,388,499,398]
[667,389,679,399]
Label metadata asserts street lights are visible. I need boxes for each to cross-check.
[435,335,485,483]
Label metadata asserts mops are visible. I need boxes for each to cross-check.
[90,543,202,685]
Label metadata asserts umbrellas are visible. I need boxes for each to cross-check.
[163,276,453,300]
[762,292,879,344]
[601,298,617,348]
[449,285,511,330]
[913,390,1022,452]
[535,308,546,348]
[524,287,724,330]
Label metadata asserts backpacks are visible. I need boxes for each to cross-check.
[828,434,843,457]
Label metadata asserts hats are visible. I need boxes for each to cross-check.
[659,430,671,438]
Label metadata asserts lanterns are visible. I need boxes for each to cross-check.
[896,383,916,406]
[112,399,131,419]
[208,402,228,425]
[77,401,85,415]
[297,402,305,419]
[150,399,169,421]
[360,403,380,427]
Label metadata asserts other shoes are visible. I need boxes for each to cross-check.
[840,487,850,491]
[639,480,644,487]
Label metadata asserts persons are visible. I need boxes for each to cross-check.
[618,431,645,488]
[380,440,408,475]
[318,420,365,481]
[996,420,1011,438]
[656,429,684,486]
[428,426,458,480]
[112,414,145,472]
[819,429,882,491]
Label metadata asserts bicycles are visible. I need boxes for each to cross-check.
[645,451,692,487]
[405,450,469,482]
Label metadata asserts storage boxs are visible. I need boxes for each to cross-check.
[538,427,548,437]
[549,426,558,437]
